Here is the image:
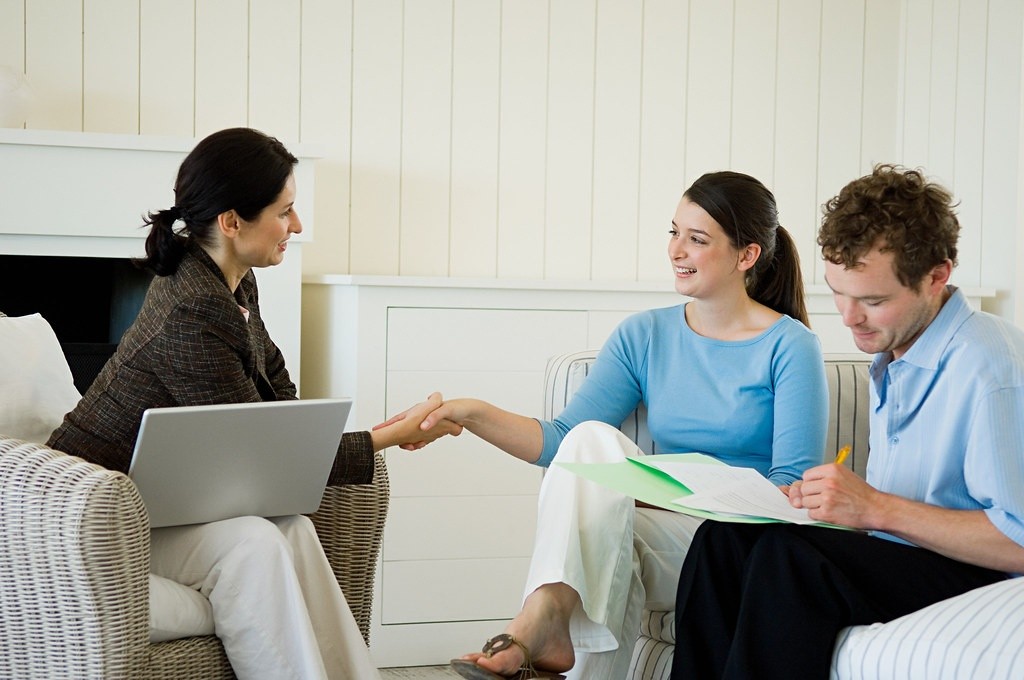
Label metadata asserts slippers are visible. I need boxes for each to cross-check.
[450,633,568,680]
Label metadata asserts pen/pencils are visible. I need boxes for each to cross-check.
[833,444,853,463]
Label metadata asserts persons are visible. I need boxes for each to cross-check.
[42,125,464,680]
[372,169,833,680]
[667,159,1024,680]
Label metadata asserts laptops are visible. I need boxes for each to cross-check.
[127,398,353,529]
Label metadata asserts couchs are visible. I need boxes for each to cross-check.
[545,349,1024,680]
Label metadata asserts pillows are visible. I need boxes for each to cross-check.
[0,311,83,445]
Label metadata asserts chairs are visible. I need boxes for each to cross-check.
[0,434,389,680]
